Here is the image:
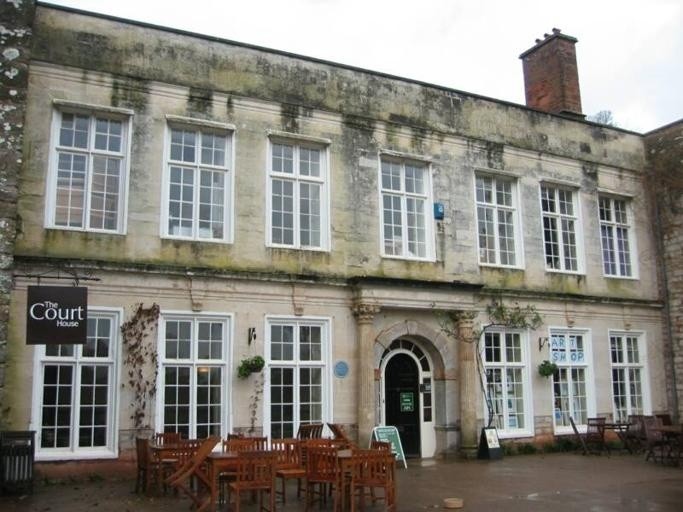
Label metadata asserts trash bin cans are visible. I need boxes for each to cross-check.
[0,430,37,494]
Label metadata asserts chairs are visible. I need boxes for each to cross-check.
[570,411,682,465]
[133,420,396,512]
